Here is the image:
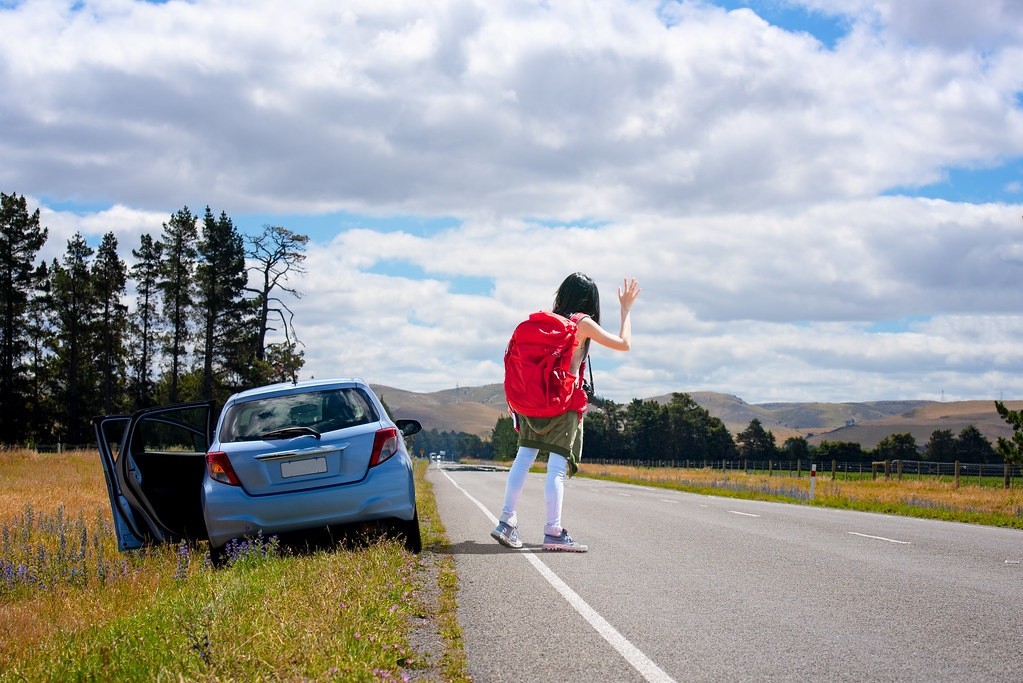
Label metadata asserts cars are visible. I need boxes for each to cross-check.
[437,455,441,459]
[93,375,424,565]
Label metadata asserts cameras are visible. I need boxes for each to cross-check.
[582,384,606,408]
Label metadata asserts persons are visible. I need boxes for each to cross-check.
[491,272,641,551]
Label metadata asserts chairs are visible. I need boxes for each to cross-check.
[248,410,278,434]
[321,390,354,421]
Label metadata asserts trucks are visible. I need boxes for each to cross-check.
[429,453,436,459]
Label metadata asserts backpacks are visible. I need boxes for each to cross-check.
[502,311,590,419]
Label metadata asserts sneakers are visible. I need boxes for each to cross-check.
[542,528,588,552]
[490,522,523,548]
